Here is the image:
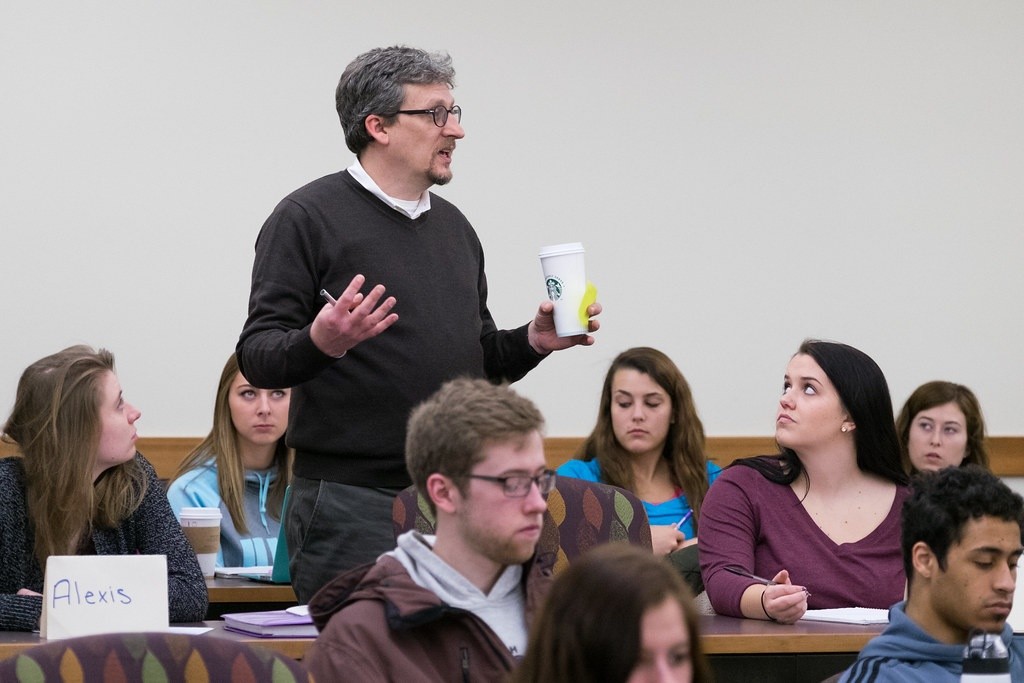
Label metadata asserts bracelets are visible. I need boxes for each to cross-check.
[761,591,776,620]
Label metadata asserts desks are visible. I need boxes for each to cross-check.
[204,577,296,603]
[1,618,889,662]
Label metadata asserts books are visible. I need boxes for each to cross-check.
[222,610,321,639]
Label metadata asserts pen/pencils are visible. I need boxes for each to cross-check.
[319,288,338,307]
[722,565,811,597]
[674,508,693,530]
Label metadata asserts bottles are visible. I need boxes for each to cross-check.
[960,633,1011,683]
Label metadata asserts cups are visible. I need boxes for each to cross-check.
[537,243,590,339]
[180,507,221,576]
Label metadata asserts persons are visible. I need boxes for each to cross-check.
[167,353,295,576]
[556,347,723,599]
[838,465,1024,683]
[237,47,601,632]
[896,381,991,477]
[515,542,716,683]
[0,345,208,631]
[698,341,911,622]
[302,378,556,683]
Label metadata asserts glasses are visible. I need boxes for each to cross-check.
[384,105,462,127]
[448,469,556,498]
